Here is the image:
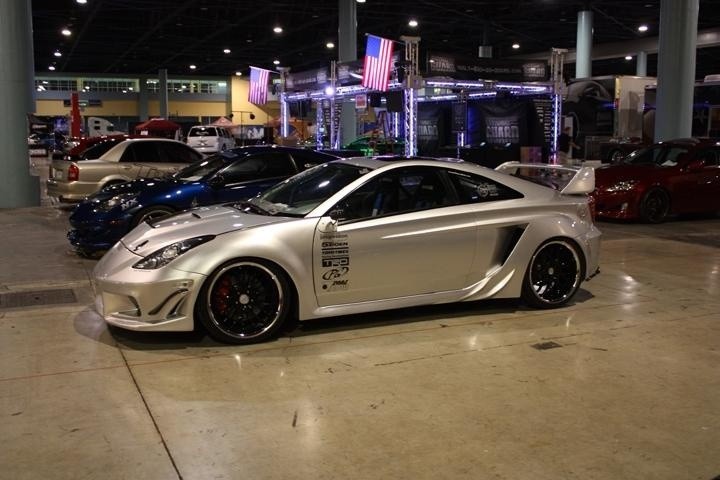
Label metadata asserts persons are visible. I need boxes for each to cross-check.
[558,126,581,165]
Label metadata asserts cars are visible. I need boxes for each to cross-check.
[340,135,405,156]
[45,133,210,203]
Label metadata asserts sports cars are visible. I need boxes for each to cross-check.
[66,144,344,260]
[91,153,603,345]
[588,138,720,223]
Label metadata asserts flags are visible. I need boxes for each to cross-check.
[361,34,394,93]
[248,66,269,105]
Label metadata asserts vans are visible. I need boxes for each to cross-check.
[186,126,236,153]
